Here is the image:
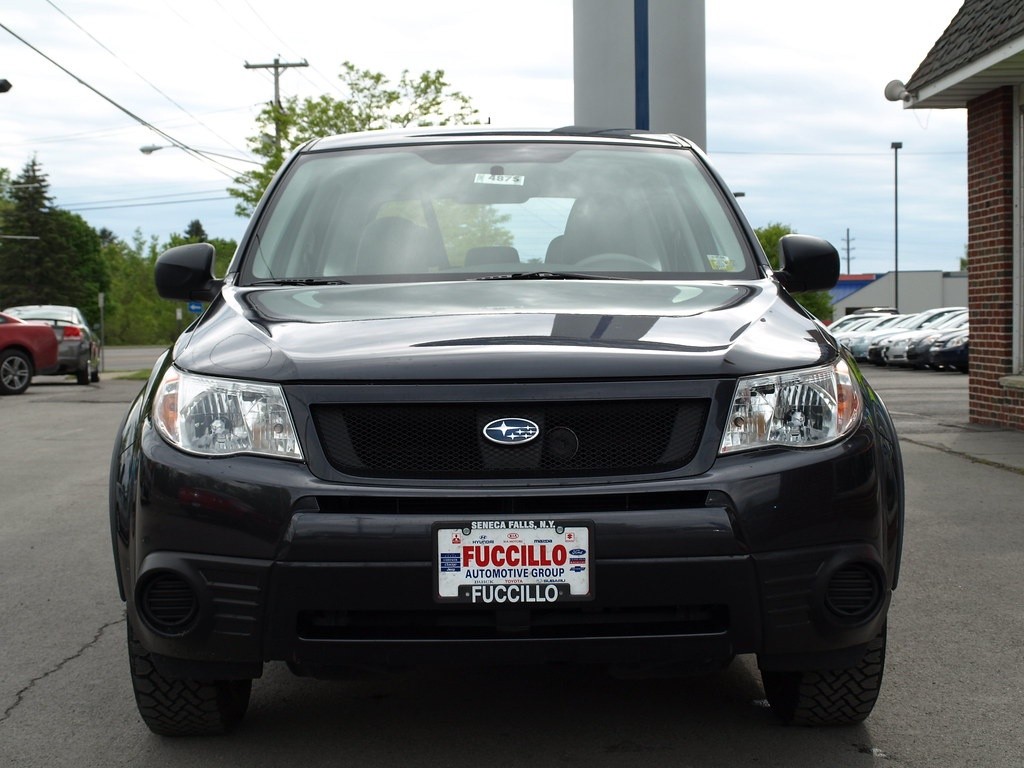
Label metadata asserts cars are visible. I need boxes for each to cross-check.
[906,313,969,370]
[0,312,58,395]
[107,125,908,725]
[928,330,969,374]
[827,312,892,334]
[881,311,969,370]
[852,307,900,314]
[848,315,916,357]
[866,307,967,367]
[834,318,877,348]
[2,305,101,384]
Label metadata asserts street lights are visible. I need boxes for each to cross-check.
[890,141,904,306]
[138,144,270,188]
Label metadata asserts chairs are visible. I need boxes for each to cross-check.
[463,245,522,269]
[335,214,434,275]
[561,192,659,272]
